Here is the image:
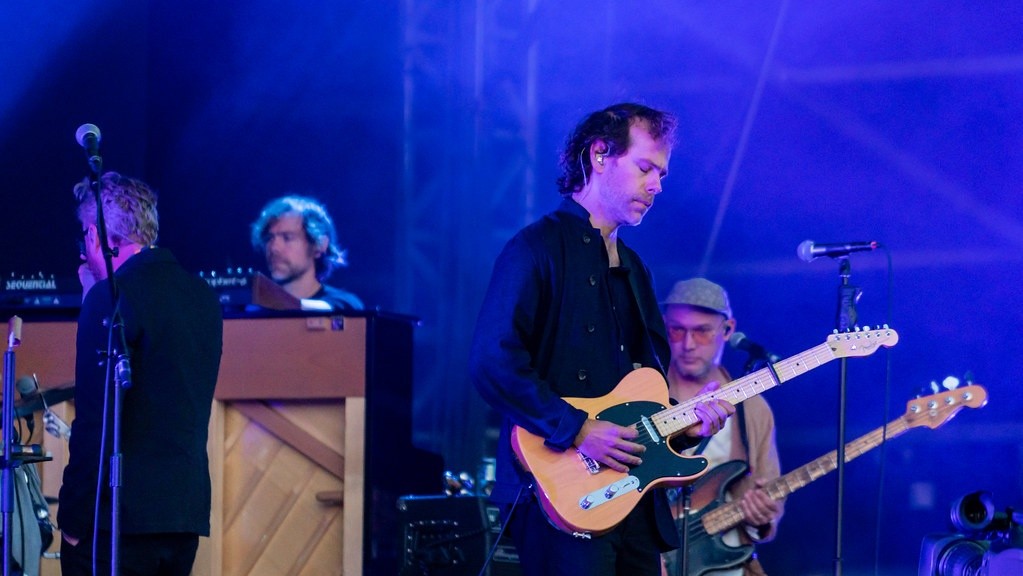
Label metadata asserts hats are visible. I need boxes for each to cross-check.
[657,278,730,320]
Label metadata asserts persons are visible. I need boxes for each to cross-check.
[244,193,364,318]
[55,170,225,576]
[474,103,736,576]
[659,277,787,576]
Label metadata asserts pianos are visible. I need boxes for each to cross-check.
[0,269,303,313]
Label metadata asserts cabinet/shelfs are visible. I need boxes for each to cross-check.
[0,307,424,576]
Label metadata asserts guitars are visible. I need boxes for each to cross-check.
[660,371,990,575]
[510,323,899,540]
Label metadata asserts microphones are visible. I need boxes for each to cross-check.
[75,123,103,171]
[729,332,782,363]
[15,375,38,434]
[797,240,881,262]
